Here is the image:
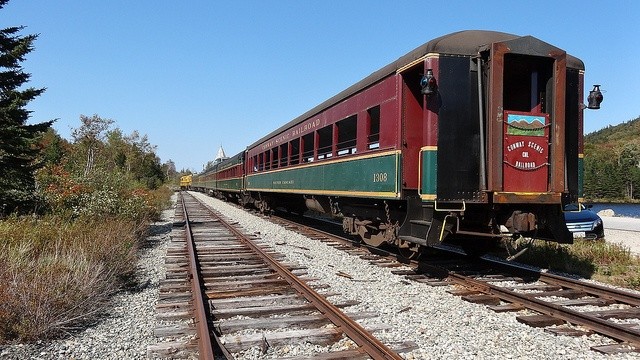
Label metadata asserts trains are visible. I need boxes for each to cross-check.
[180,30,603,260]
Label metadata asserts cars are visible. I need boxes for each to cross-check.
[565,203,604,240]
